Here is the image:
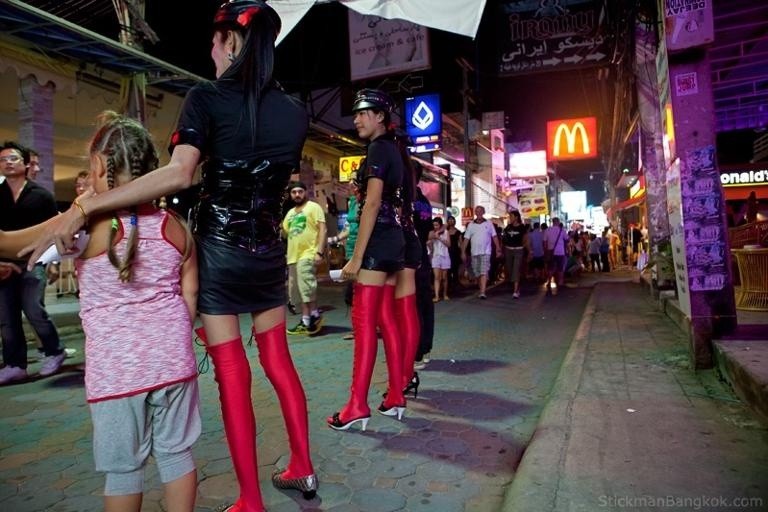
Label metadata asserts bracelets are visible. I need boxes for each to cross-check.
[73,199,89,228]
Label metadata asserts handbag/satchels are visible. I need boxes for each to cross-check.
[543,248,554,265]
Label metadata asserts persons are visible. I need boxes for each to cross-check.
[75,171,90,196]
[0,148,77,371]
[326,101,407,431]
[280,182,363,335]
[410,158,435,368]
[385,128,421,399]
[17,1,319,512]
[426,206,648,299]
[0,141,67,385]
[0,110,199,512]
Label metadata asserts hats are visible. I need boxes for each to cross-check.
[351,87,392,114]
[285,180,308,192]
[210,0,283,42]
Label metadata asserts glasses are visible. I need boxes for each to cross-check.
[0,154,25,163]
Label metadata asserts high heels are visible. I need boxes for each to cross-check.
[376,401,408,422]
[381,371,421,400]
[270,467,320,502]
[327,411,372,434]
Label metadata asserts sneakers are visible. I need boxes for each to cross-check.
[423,352,432,364]
[38,350,68,377]
[305,314,325,335]
[414,359,427,370]
[0,364,28,385]
[285,318,305,335]
